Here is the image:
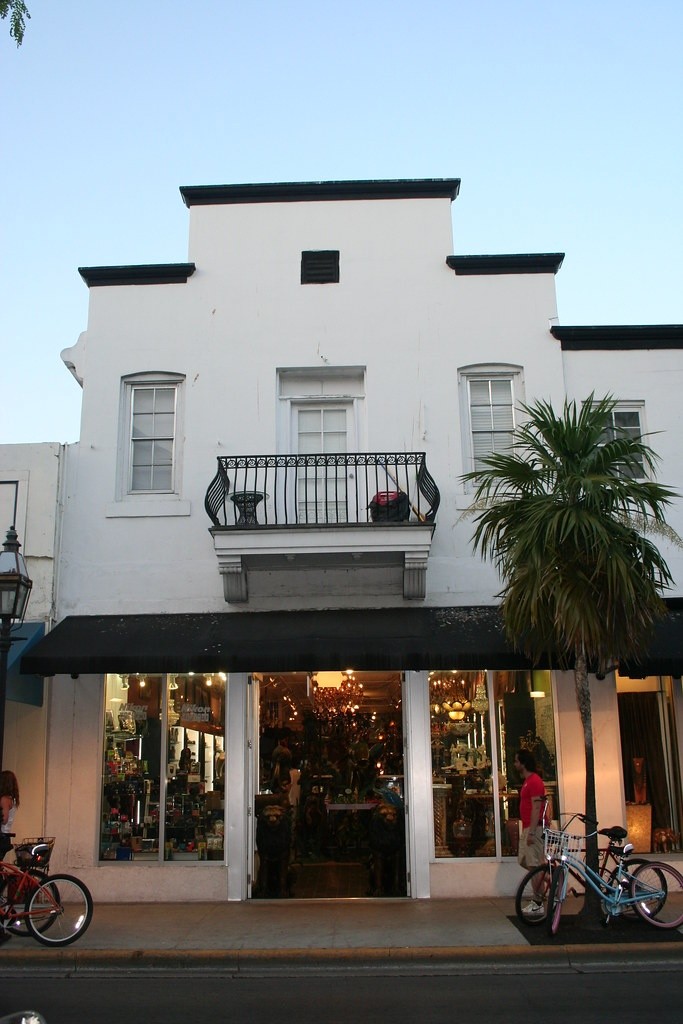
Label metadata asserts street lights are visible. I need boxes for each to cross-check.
[0,525,40,768]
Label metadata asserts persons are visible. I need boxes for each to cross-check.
[0,769,19,861]
[179,748,192,773]
[521,728,550,777]
[514,749,558,915]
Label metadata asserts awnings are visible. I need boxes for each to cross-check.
[0,622,45,708]
[19,599,683,674]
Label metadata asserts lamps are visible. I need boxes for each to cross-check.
[206,677,213,686]
[120,675,129,689]
[136,675,146,687]
[169,675,178,690]
[428,672,488,734]
[310,671,364,714]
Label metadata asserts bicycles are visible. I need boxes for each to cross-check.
[0,828,94,947]
[515,793,683,935]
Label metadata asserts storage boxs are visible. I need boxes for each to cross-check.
[187,773,200,782]
[142,839,155,850]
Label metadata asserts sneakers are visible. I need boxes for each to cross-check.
[522,900,544,915]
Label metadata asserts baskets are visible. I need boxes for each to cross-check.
[15,837,56,866]
[544,829,583,863]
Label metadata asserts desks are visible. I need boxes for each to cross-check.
[224,490,270,524]
[326,802,379,850]
